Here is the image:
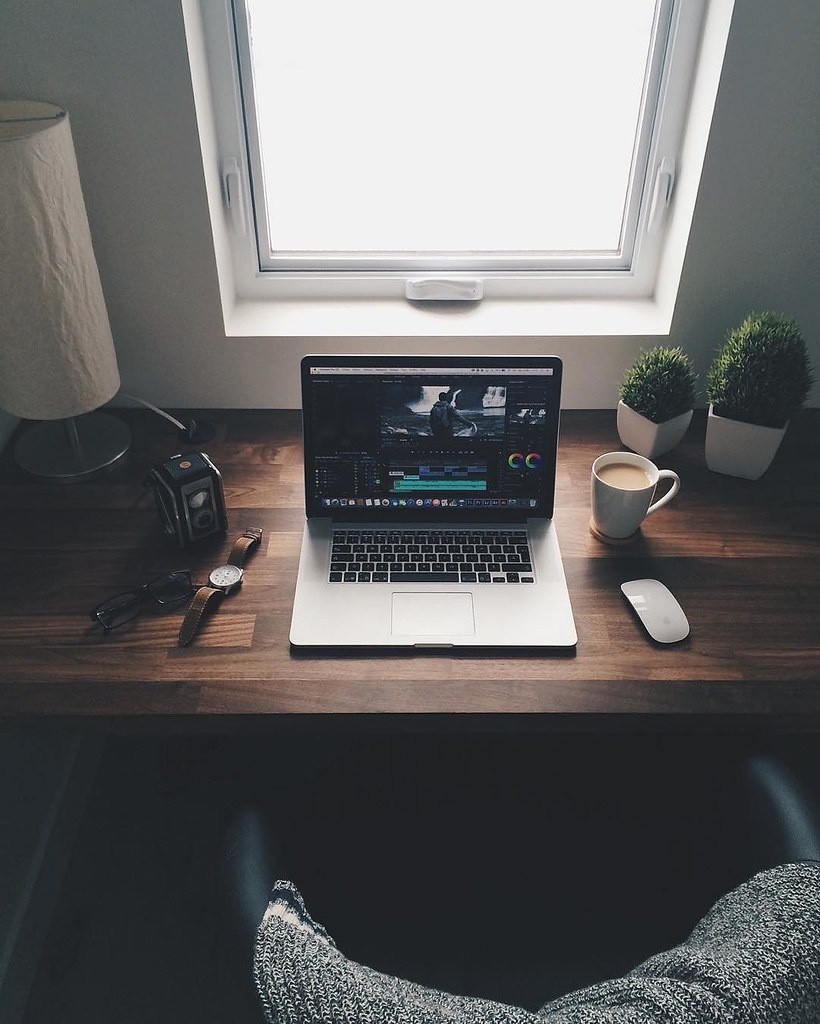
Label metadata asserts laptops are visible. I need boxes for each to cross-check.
[290,353,578,651]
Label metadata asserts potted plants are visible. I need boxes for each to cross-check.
[704,308,816,483]
[612,342,702,460]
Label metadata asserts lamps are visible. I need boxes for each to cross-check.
[0,96,137,479]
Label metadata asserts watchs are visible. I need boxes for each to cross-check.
[179,526,263,648]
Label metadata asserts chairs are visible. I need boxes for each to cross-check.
[221,744,820,1024]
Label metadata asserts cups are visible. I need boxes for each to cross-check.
[590,452,680,539]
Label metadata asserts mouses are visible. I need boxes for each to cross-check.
[620,578,690,644]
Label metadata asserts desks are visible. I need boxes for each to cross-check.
[0,405,820,746]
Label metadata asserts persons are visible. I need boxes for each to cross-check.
[430,392,477,450]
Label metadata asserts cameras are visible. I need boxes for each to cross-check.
[150,450,228,549]
